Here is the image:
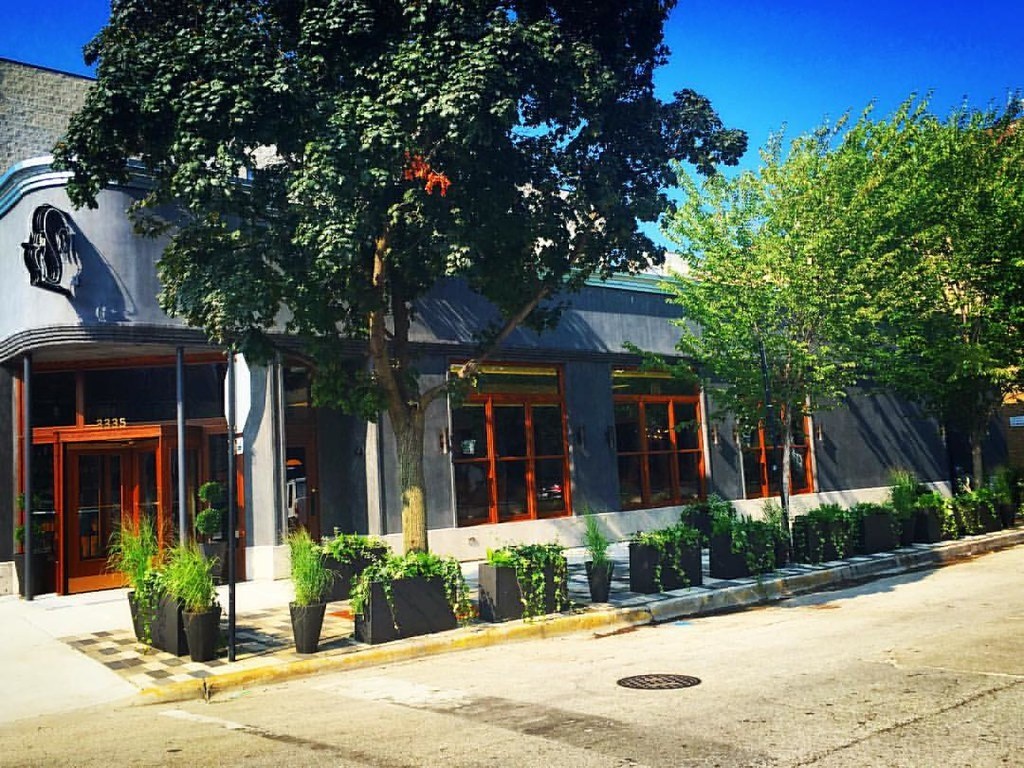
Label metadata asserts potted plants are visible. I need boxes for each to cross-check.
[103,479,228,662]
[351,546,480,647]
[577,501,615,606]
[276,520,343,655]
[476,541,588,624]
[628,461,1024,596]
[315,527,395,606]
[13,490,43,594]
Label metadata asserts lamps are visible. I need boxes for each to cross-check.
[816,423,823,441]
[439,427,448,454]
[634,406,670,440]
[605,426,614,449]
[939,426,946,441]
[732,421,740,445]
[711,422,720,446]
[576,424,586,450]
